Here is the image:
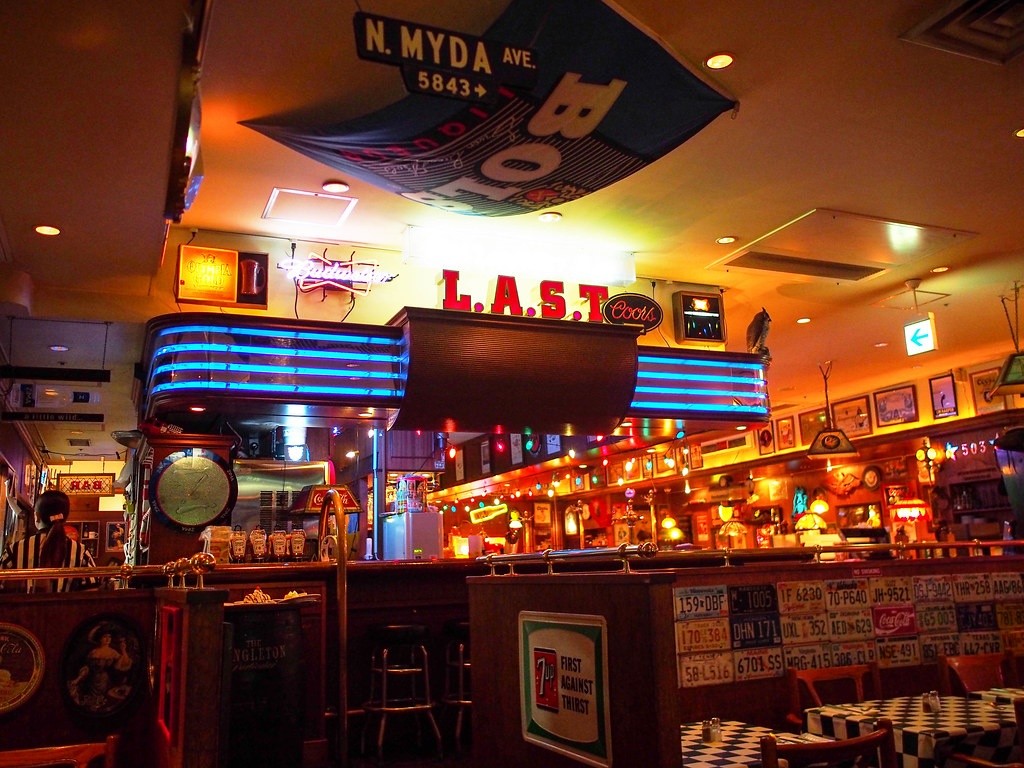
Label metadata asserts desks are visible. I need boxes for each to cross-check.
[803,695,1017,768]
[968,688,1024,703]
[681,718,862,768]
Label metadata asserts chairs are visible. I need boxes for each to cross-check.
[762,717,899,768]
[938,648,1021,697]
[783,659,884,732]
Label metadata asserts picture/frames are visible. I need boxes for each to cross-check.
[105,521,128,552]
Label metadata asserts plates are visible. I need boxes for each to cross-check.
[233,594,321,604]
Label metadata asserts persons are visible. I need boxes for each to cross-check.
[0,490,100,594]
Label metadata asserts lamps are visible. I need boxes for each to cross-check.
[505,440,938,554]
[806,361,859,460]
[990,280,1023,396]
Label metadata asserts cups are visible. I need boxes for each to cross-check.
[89,532,98,538]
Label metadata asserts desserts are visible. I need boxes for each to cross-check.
[243,587,271,603]
[284,590,307,599]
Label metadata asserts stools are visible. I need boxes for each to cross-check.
[438,620,473,748]
[363,620,440,752]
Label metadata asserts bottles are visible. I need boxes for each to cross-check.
[89,542,94,555]
[230,529,306,562]
[83,524,89,537]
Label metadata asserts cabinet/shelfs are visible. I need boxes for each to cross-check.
[65,520,101,558]
[946,469,1009,525]
